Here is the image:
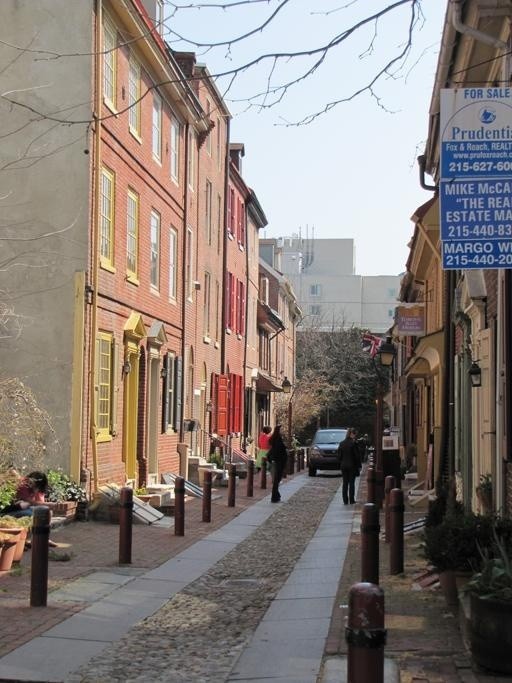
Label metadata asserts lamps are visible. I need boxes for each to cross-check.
[378,336,398,367]
[282,377,292,393]
[207,400,214,412]
[122,360,130,376]
[160,367,168,379]
[468,358,482,387]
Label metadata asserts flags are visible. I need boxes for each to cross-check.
[362,333,382,361]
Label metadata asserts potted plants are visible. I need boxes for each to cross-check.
[0,514,32,571]
[420,471,512,677]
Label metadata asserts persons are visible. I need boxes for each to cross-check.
[337,428,362,505]
[356,434,369,463]
[0,472,58,547]
[255,425,288,502]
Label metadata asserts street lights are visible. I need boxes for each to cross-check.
[281,374,297,448]
[371,334,398,509]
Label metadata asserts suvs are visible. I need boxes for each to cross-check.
[306,426,361,477]
